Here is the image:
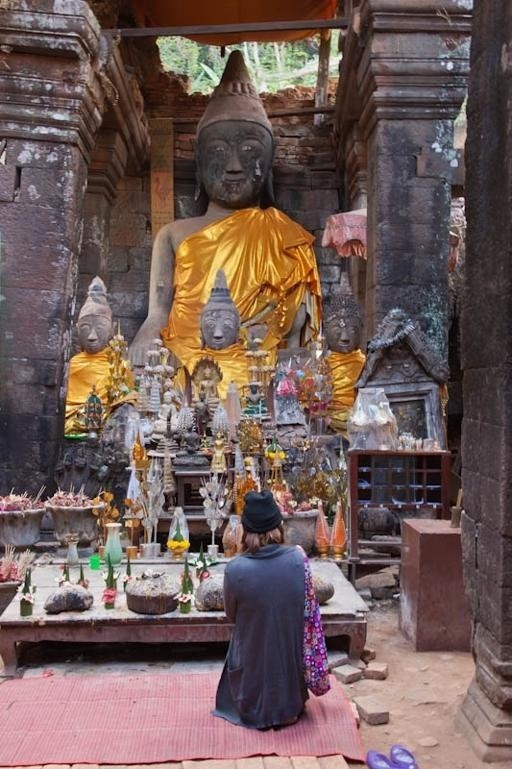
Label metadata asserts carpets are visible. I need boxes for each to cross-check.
[0,672,367,766]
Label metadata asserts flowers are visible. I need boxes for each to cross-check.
[276,492,313,513]
[0,545,18,581]
[0,484,99,511]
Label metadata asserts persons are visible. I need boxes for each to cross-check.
[63,302,122,434]
[126,115,327,374]
[132,367,271,476]
[309,303,369,435]
[184,301,254,400]
[210,487,311,735]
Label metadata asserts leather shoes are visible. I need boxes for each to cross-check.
[367,745,419,768]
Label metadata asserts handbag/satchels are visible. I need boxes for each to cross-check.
[303,591,330,697]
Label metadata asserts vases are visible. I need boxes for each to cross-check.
[0,503,106,549]
[0,583,21,613]
[282,510,318,555]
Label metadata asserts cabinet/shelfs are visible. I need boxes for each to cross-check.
[347,449,452,581]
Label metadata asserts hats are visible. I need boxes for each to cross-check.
[241,490,282,533]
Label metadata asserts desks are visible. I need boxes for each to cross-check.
[0,552,368,665]
